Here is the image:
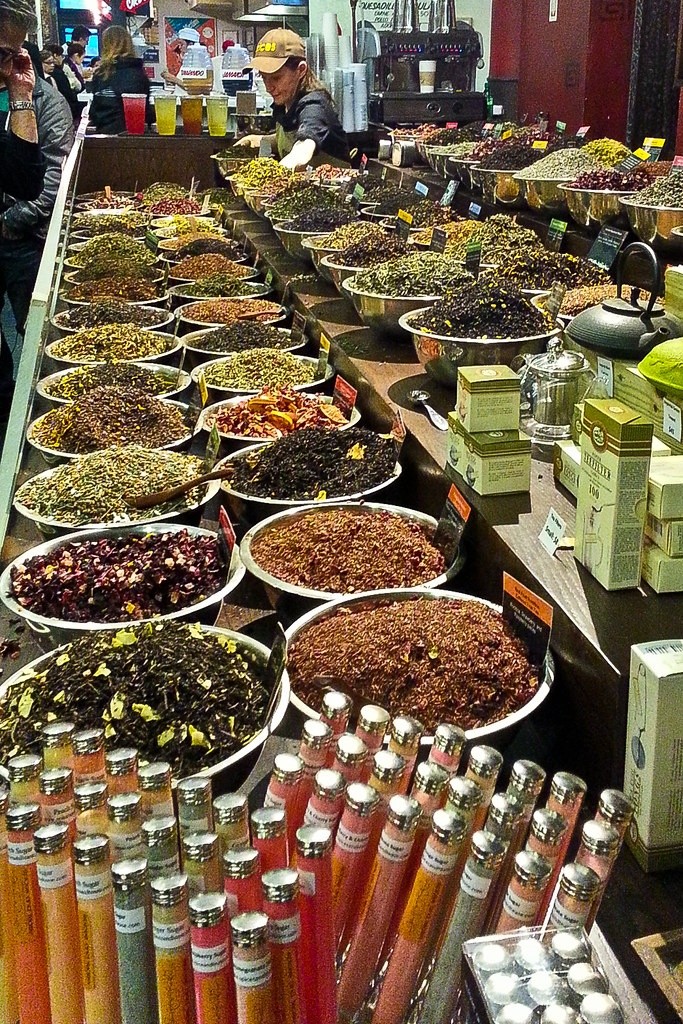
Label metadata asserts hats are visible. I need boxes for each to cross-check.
[223,39,235,50]
[177,28,200,42]
[241,28,307,73]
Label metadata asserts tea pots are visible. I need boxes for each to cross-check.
[562,241,683,374]
[508,336,609,442]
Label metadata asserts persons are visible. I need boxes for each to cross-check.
[0,0,99,450]
[235,26,350,169]
[160,27,236,95]
[84,25,150,137]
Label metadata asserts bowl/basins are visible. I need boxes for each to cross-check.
[1,129,683,787]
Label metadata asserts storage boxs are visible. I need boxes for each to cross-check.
[611,638,683,871]
[554,397,683,593]
[447,364,534,496]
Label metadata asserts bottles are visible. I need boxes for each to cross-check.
[221,44,254,95]
[182,43,214,95]
[484,83,493,120]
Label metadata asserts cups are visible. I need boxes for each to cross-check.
[206,96,229,136]
[310,14,368,133]
[180,95,204,136]
[419,60,436,93]
[154,94,177,136]
[122,93,147,136]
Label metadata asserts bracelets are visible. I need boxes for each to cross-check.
[9,102,35,110]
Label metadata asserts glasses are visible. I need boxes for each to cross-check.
[43,61,55,65]
[3,51,21,64]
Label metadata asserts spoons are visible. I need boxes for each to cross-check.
[406,390,450,432]
